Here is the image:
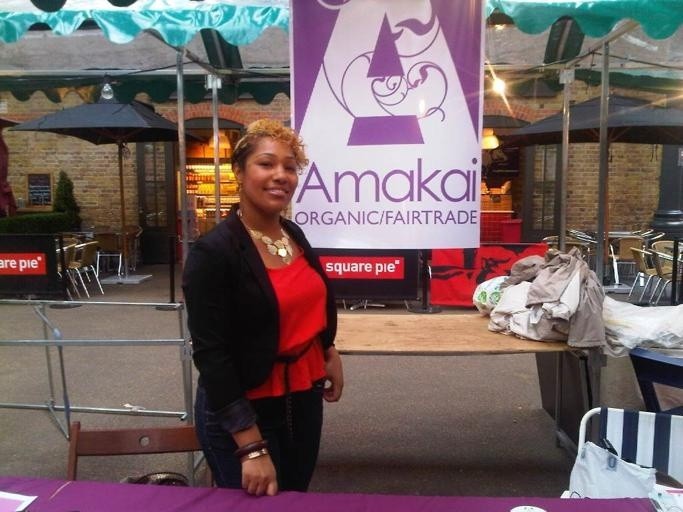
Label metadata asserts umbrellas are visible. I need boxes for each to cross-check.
[7,96,204,277]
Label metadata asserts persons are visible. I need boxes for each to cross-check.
[184,119,344,497]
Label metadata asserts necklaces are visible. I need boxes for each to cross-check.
[231,210,291,266]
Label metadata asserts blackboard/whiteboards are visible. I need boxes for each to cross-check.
[25,171,55,210]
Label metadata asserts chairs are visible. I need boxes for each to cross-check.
[55,223,143,301]
[541,226,683,306]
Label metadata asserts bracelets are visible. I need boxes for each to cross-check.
[231,437,268,462]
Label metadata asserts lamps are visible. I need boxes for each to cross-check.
[482,127,499,150]
[207,129,230,150]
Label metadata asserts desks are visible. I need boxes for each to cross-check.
[335,312,608,460]
